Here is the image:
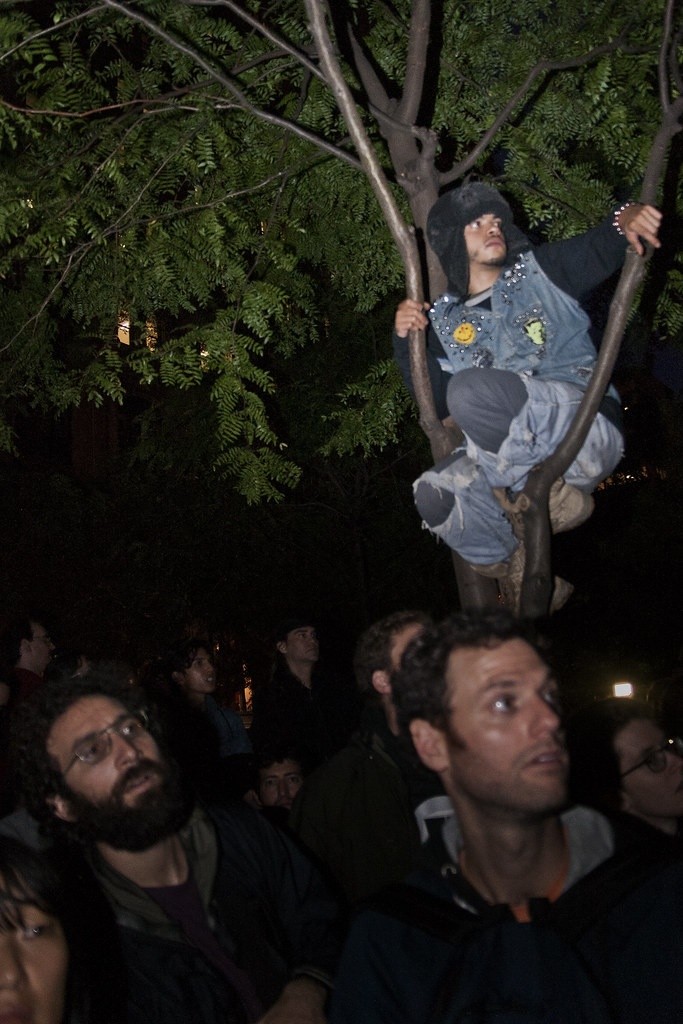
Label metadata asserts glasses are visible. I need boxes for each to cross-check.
[57,706,149,781]
[27,635,51,644]
[619,737,683,784]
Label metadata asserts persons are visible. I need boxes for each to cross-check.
[391,180,662,621]
[323,611,683,1024]
[569,698,682,837]
[296,608,448,892]
[244,613,349,768]
[0,614,314,812]
[0,833,73,1024]
[11,664,338,1024]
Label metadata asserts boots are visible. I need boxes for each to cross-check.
[469,541,572,621]
[548,474,595,534]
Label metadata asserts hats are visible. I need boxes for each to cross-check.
[425,182,532,305]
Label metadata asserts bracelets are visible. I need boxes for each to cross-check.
[612,202,641,236]
